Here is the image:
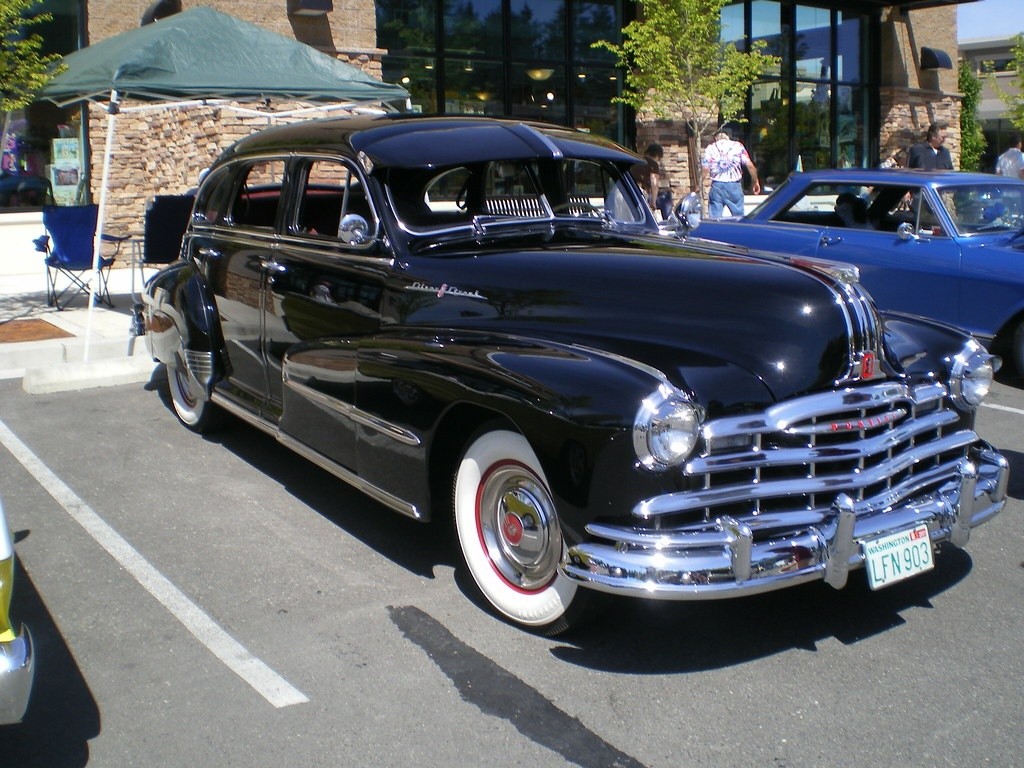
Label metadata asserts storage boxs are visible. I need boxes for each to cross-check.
[45,138,80,205]
[800,111,856,171]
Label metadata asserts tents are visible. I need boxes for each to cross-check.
[31,4,411,363]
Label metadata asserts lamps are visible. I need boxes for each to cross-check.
[141,0,180,27]
[286,0,333,17]
[921,46,953,71]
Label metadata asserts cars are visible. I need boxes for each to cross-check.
[676,167,1024,382]
[129,112,1011,641]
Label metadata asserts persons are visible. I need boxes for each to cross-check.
[994,138,1023,180]
[633,143,665,211]
[910,117,949,170]
[696,128,762,219]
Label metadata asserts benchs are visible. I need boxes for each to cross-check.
[487,194,595,216]
[786,211,898,236]
[238,194,434,237]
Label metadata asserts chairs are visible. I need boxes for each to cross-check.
[35,205,130,308]
[131,194,198,308]
